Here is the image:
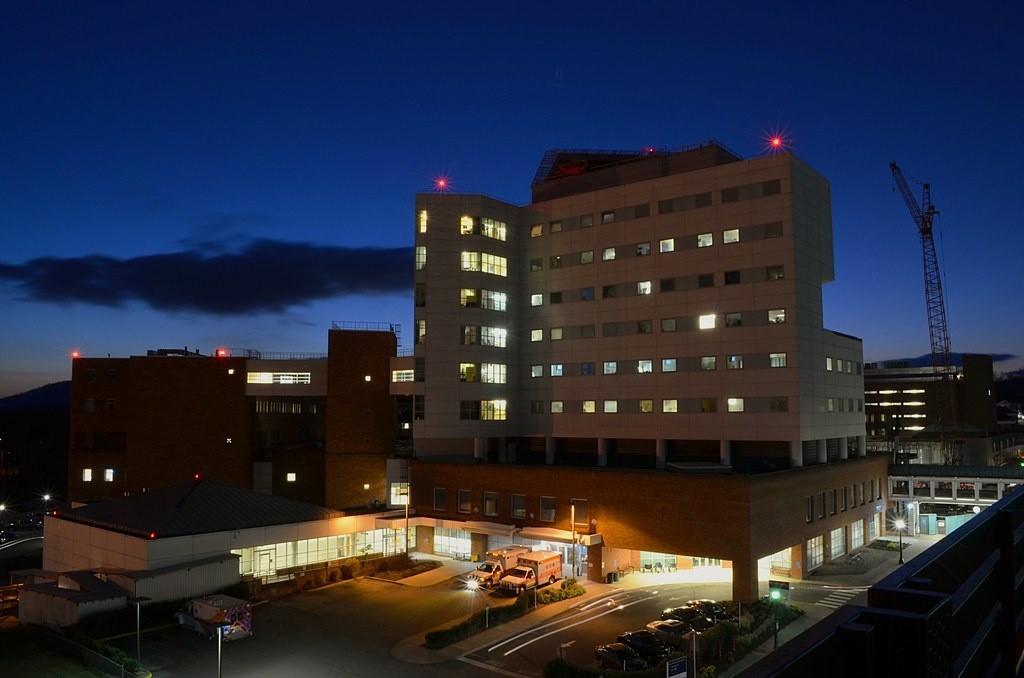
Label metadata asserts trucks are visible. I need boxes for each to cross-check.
[172,592,253,642]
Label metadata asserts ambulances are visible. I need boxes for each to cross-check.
[467,544,528,589]
[498,550,562,596]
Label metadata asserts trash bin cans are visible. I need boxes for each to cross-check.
[471,552,478,562]
[607,572,614,584]
[613,571,619,582]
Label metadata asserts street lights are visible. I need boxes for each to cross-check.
[569,502,577,581]
[770,590,781,651]
[908,501,919,539]
[895,519,905,564]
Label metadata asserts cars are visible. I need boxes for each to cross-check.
[618,629,680,666]
[662,605,714,635]
[646,619,701,651]
[594,641,647,671]
[686,598,735,624]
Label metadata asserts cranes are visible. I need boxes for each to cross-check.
[888,157,968,467]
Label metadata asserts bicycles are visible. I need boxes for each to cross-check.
[845,553,865,565]
